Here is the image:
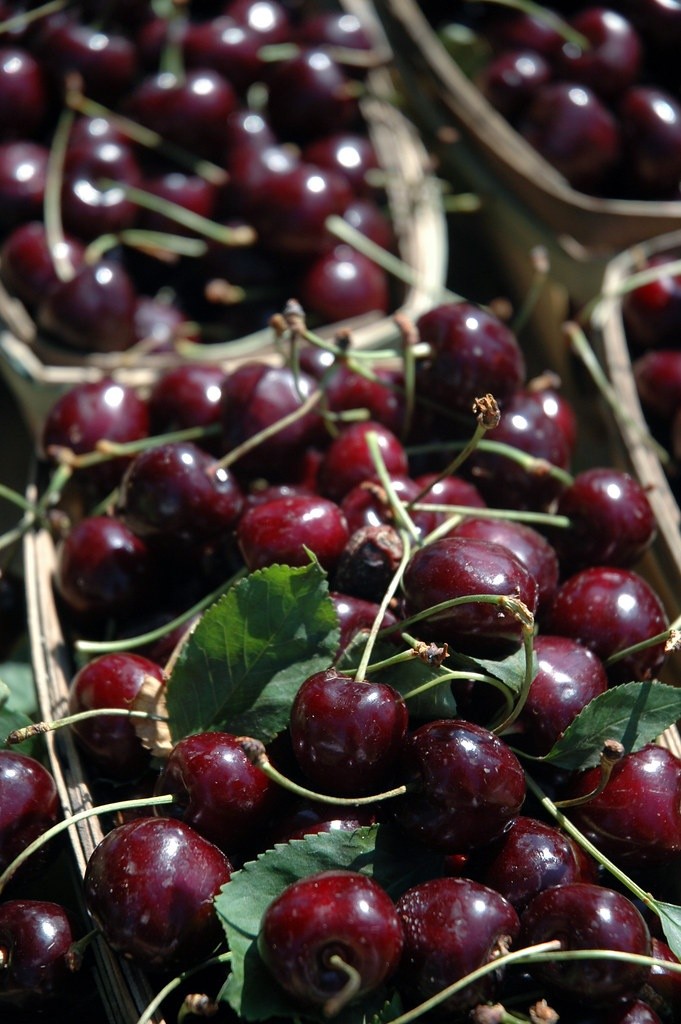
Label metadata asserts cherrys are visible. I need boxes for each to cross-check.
[0,0,681,1024]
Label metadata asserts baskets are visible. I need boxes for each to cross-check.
[0,0,681,1024]
